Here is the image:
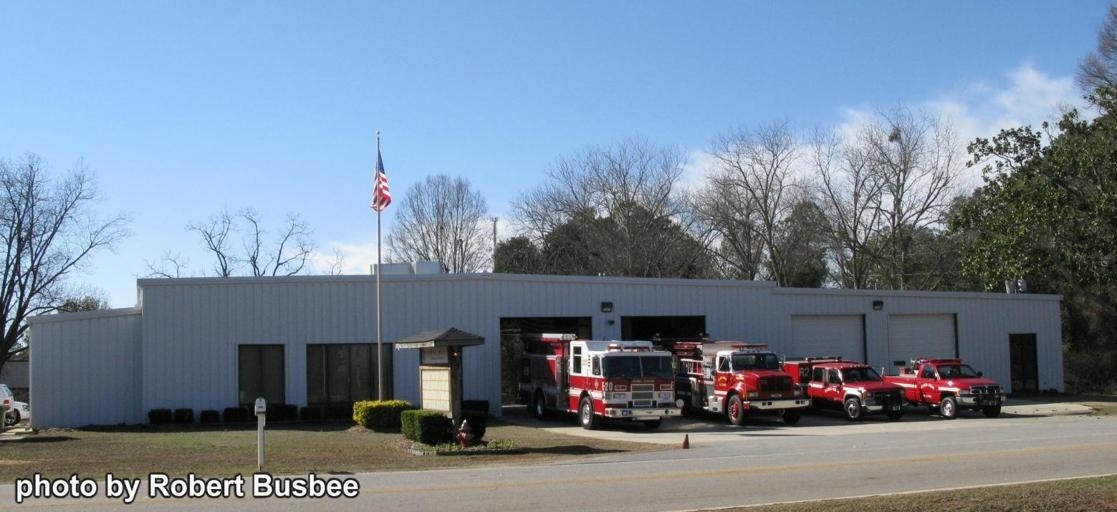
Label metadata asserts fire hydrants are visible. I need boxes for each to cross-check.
[456,420,475,449]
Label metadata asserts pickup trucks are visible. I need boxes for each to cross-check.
[780,357,909,421]
[881,359,1007,419]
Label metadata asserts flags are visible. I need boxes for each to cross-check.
[369,145,391,213]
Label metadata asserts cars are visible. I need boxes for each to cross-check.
[0,384,30,432]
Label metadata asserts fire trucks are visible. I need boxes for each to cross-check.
[648,333,812,426]
[515,331,684,430]
